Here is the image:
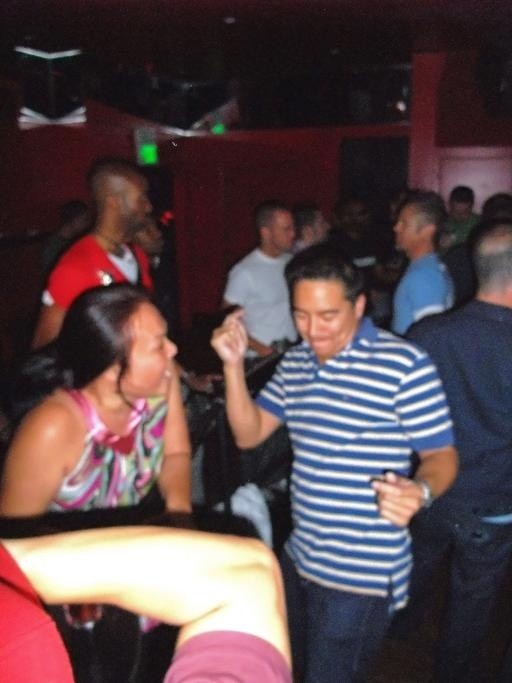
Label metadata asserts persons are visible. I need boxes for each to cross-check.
[14,152,182,407]
[3,524,296,683]
[1,285,193,683]
[222,177,512,369]
[392,229,511,683]
[209,247,461,682]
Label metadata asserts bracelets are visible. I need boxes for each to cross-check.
[414,476,435,510]
